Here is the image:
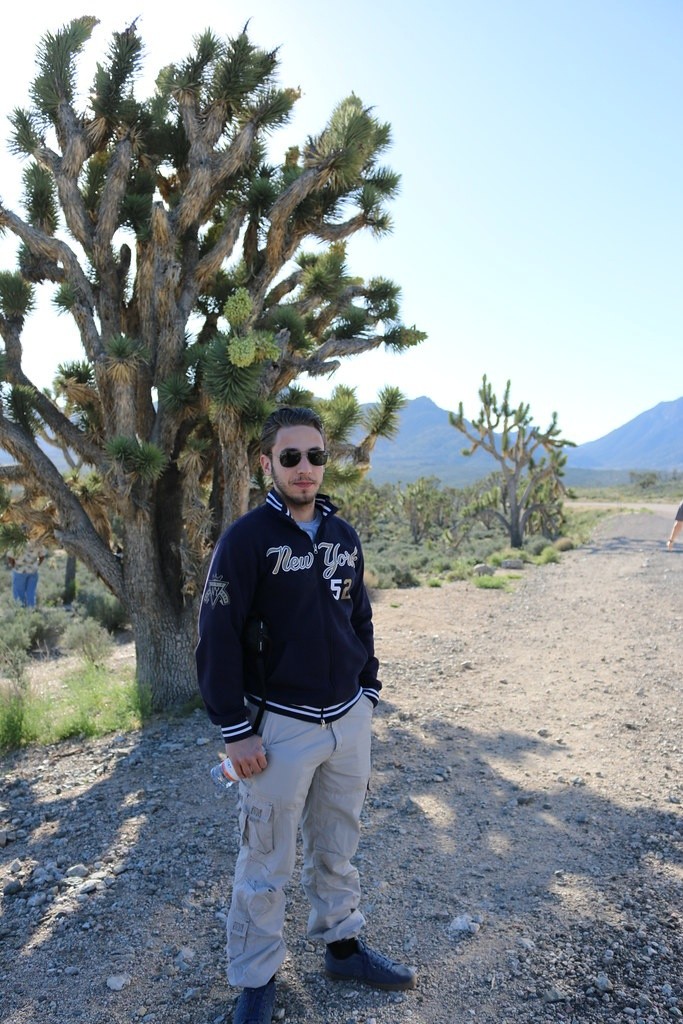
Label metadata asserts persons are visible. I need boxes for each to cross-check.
[194,409,413,1024]
[6,523,45,608]
[667,500,683,550]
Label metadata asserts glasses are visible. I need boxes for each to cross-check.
[268,449,329,468]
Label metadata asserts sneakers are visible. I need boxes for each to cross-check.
[325,938,416,992]
[233,975,277,1024]
